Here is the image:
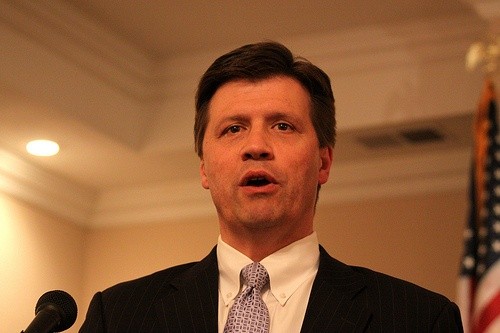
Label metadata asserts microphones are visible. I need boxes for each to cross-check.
[20,289,78,333]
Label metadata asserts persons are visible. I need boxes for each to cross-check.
[80,42,465,333]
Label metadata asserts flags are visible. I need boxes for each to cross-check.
[456,81,500,333]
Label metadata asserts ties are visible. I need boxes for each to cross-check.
[220,263,273,333]
